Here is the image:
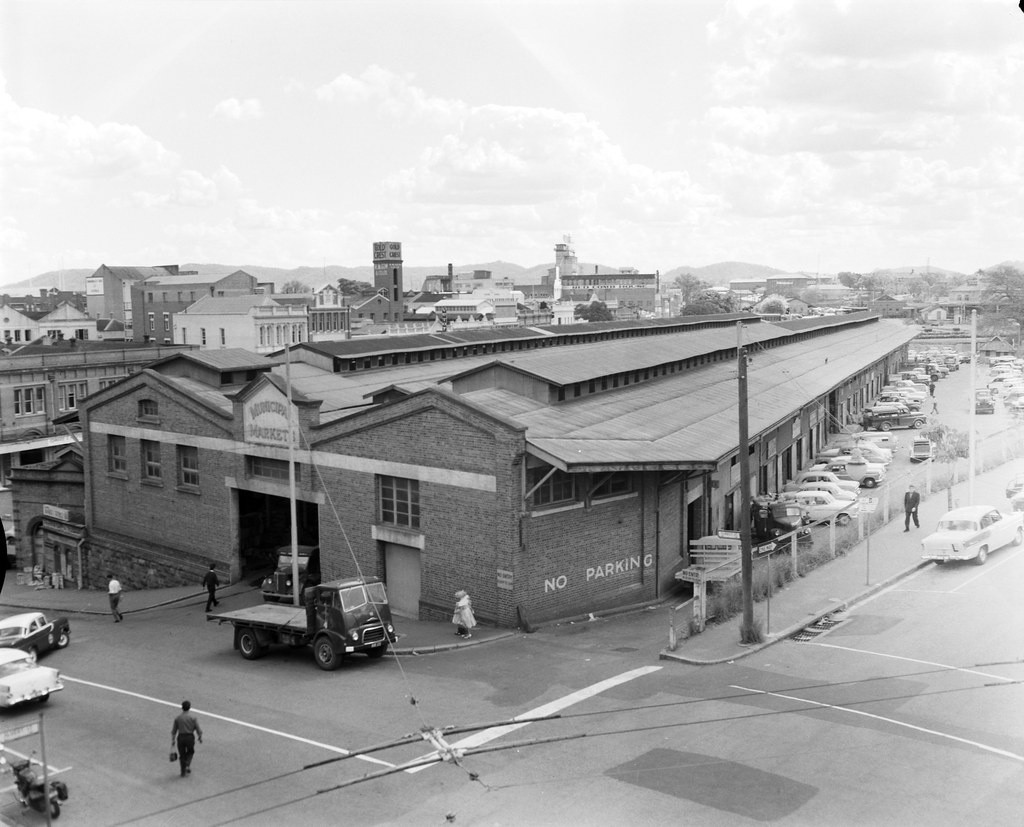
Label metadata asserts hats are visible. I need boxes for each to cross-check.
[107,575,113,578]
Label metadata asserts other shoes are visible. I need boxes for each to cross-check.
[455,632,465,635]
[461,634,471,639]
[113,619,119,623]
[205,609,212,612]
[119,615,123,620]
[916,525,920,528]
[214,600,219,607]
[904,529,909,532]
[181,767,191,777]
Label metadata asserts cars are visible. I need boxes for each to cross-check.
[910,439,935,461]
[784,492,858,525]
[0,613,71,663]
[811,432,892,488]
[909,346,969,377]
[0,648,63,710]
[795,482,856,503]
[874,363,941,412]
[781,473,860,491]
[920,505,1024,565]
[1006,474,1024,510]
[990,355,1024,409]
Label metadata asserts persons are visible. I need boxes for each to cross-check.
[202,564,220,612]
[452,591,477,640]
[930,396,939,414]
[903,485,920,532]
[751,496,763,523]
[106,574,123,623]
[863,410,869,431]
[929,383,936,396]
[170,700,203,777]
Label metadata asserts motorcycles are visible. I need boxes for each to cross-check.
[0,749,68,819]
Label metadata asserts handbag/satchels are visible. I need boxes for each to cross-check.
[169,745,177,762]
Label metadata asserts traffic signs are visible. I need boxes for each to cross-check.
[757,540,778,555]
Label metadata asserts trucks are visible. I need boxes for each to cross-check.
[207,574,396,670]
[674,530,753,588]
[975,389,995,414]
[757,501,813,551]
[261,546,323,606]
[864,406,927,431]
